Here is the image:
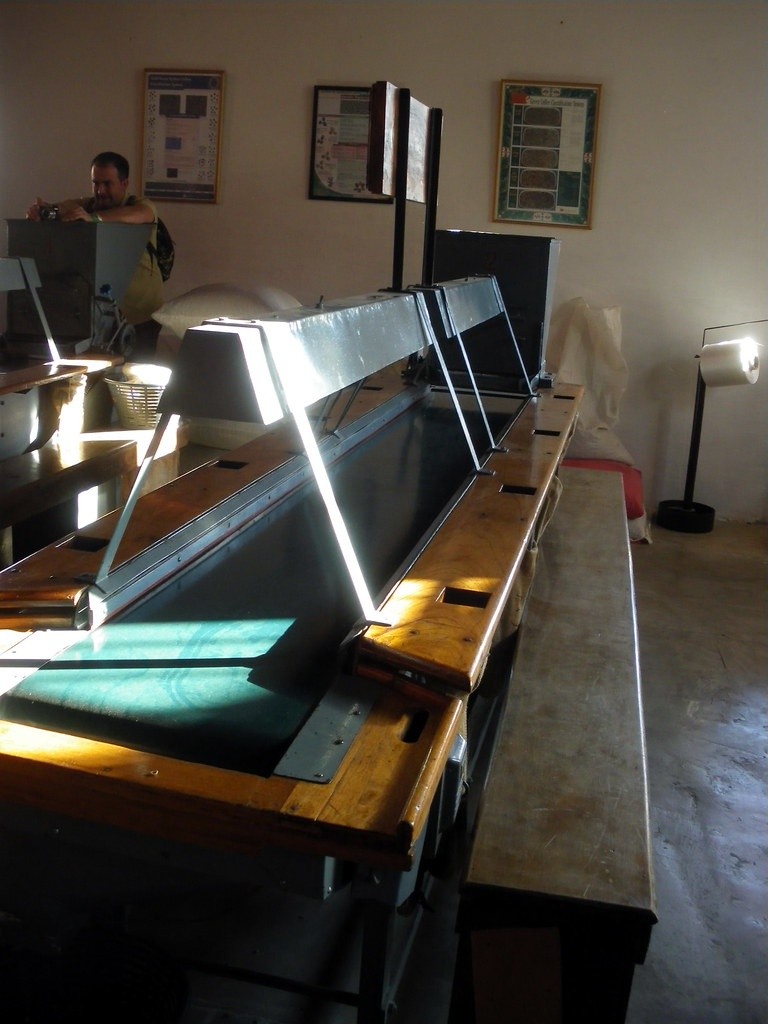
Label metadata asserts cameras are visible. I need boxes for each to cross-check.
[40,205,59,220]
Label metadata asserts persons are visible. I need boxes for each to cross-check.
[26,151,166,367]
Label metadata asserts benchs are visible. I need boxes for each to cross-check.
[0,420,190,531]
[447,466,659,1024]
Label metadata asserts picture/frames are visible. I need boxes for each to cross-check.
[491,79,603,230]
[138,69,225,205]
[309,85,394,206]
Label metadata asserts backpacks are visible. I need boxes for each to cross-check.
[85,195,175,281]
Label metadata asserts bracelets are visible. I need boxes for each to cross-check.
[91,212,103,222]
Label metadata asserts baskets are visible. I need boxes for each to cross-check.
[104,373,164,429]
